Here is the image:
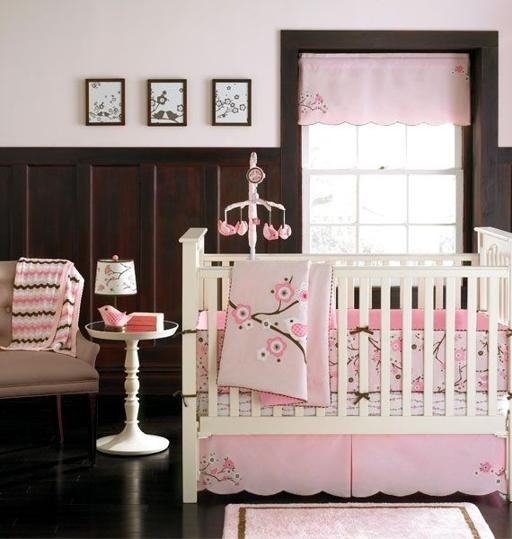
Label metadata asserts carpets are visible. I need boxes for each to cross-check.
[222,499,496,539]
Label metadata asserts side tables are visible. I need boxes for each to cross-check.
[82,314,182,459]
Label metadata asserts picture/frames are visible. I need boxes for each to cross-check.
[85,79,125,126]
[212,78,252,126]
[147,79,187,126]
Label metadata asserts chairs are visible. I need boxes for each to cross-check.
[0,259,102,458]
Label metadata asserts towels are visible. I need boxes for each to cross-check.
[0,255,84,357]
[215,254,336,411]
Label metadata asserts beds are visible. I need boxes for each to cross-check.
[176,225,512,505]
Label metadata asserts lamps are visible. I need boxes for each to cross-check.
[94,253,138,314]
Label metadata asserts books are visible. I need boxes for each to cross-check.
[128,312,164,326]
[125,325,166,332]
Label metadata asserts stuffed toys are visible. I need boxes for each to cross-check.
[98,304,130,327]
